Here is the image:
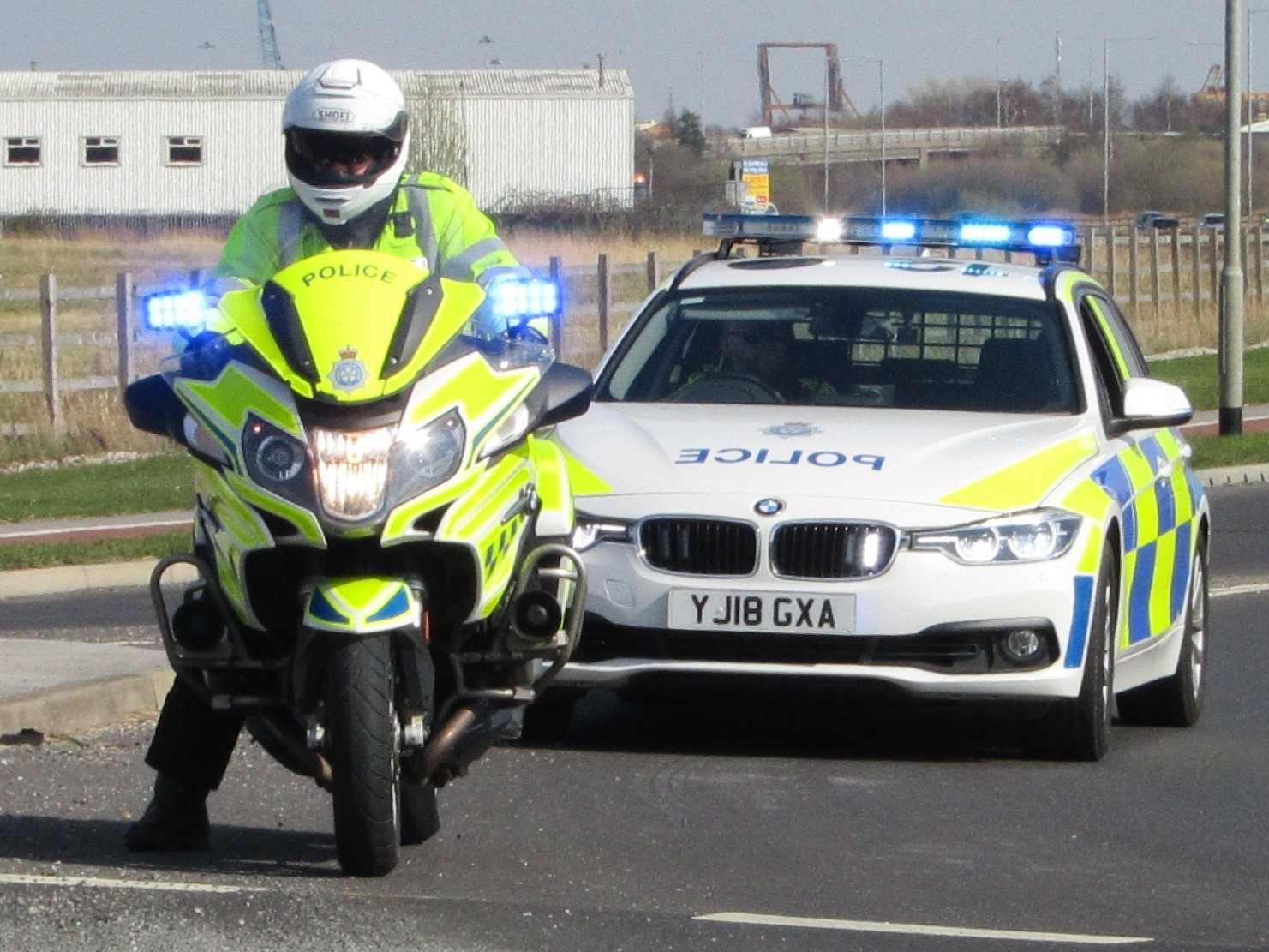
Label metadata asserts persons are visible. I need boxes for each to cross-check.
[686,301,821,405]
[124,59,549,851]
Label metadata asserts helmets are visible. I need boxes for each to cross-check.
[282,58,413,225]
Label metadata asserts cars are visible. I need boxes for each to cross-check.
[1134,210,1180,237]
[1199,212,1225,236]
[518,208,1216,763]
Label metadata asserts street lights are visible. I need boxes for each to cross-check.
[1101,34,1161,234]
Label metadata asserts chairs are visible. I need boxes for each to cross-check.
[783,319,1061,407]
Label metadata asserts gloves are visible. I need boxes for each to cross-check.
[489,326,548,370]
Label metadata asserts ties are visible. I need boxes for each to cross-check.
[721,358,735,375]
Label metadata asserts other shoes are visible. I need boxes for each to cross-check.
[514,660,537,687]
[122,797,211,853]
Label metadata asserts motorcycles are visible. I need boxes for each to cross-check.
[122,250,591,880]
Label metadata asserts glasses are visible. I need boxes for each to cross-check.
[720,330,746,339]
[306,144,373,164]
